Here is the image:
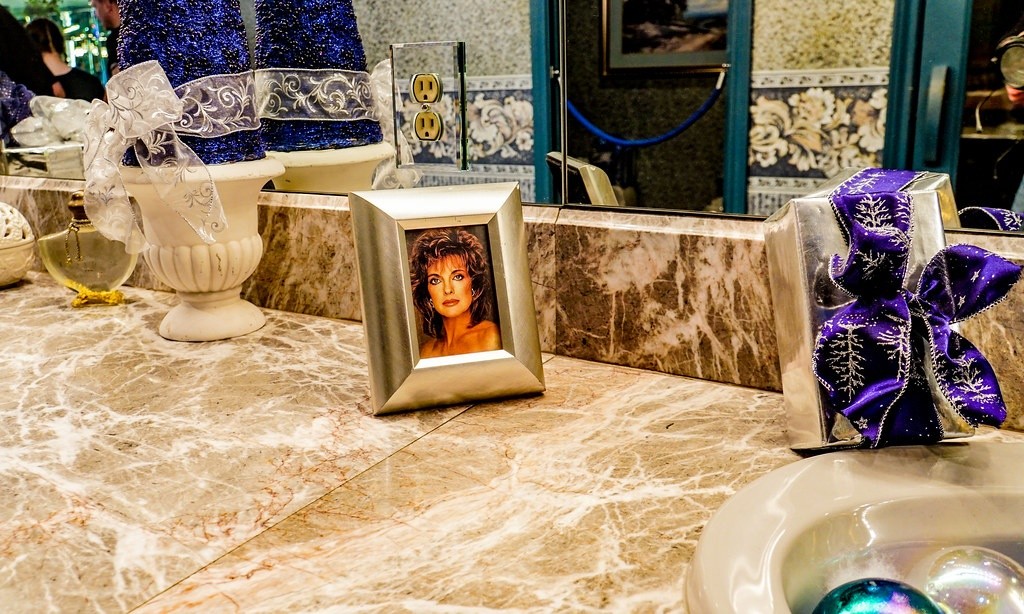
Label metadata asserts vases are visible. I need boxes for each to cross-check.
[263,141,395,195]
[119,156,287,343]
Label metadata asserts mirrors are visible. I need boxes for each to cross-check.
[0,0,1024,236]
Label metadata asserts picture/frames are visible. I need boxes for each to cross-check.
[348,181,548,416]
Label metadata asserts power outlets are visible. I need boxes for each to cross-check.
[389,40,468,171]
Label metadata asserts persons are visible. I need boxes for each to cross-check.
[25,19,106,103]
[89,0,121,79]
[408,226,503,359]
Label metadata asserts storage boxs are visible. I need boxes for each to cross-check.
[817,164,964,229]
[761,189,1024,449]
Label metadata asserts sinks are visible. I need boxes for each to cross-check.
[683,438,1024,614]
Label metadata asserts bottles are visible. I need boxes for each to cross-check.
[36,191,139,308]
